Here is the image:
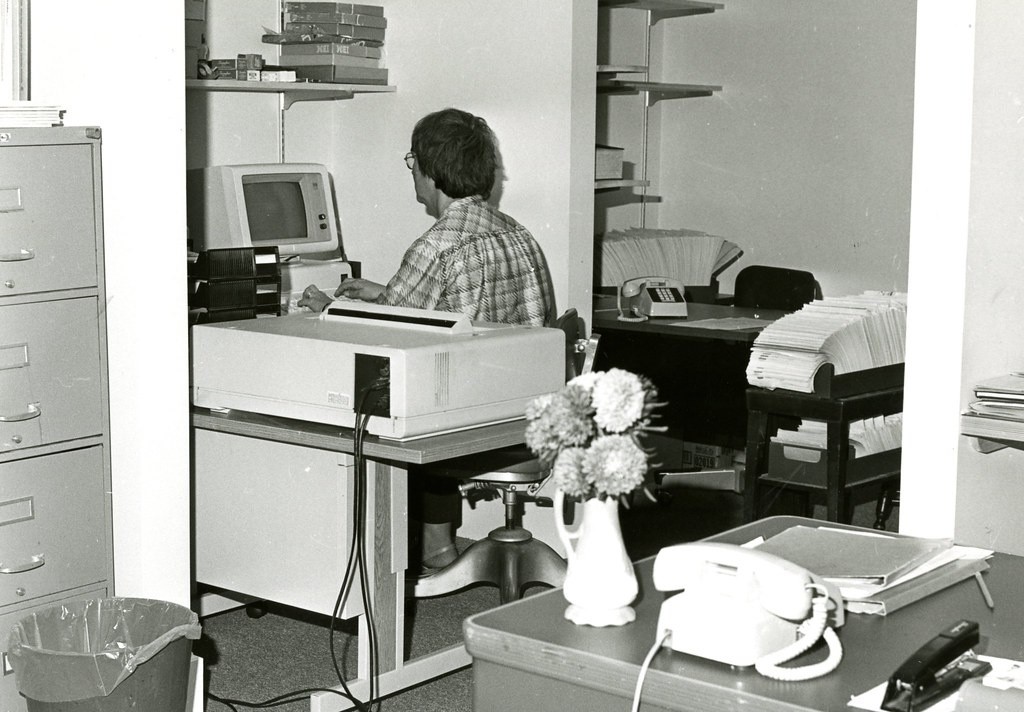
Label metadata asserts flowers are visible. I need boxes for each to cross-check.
[528,366,669,509]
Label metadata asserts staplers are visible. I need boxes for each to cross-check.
[876,620,996,711]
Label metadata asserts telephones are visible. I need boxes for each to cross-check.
[618,274,688,319]
[649,539,846,682]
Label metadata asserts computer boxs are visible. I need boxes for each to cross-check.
[255,260,353,304]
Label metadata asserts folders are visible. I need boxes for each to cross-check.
[827,555,993,614]
[757,525,954,590]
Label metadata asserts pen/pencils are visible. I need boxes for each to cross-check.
[973,571,992,609]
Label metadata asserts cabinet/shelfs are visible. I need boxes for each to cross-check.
[745,379,903,524]
[0,126,115,712]
[593,0,724,190]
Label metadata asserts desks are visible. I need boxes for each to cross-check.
[462,513,1024,712]
[188,407,537,711]
[593,295,794,534]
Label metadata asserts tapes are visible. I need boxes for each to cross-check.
[199,63,213,76]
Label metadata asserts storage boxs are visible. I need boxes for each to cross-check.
[648,434,744,471]
[185,0,298,83]
[261,1,389,85]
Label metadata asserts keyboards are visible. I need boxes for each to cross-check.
[279,289,364,315]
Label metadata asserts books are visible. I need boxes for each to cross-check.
[968,370,1024,424]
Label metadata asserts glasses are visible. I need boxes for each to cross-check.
[403,152,418,170]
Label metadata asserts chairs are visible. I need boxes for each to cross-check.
[403,308,602,606]
[737,265,819,312]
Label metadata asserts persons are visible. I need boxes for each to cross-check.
[298,108,555,576]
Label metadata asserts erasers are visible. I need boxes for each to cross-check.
[10,597,200,710]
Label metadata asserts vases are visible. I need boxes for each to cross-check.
[553,484,639,625]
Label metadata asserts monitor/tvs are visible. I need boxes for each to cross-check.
[186,164,338,268]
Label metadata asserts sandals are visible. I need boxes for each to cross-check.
[409,543,457,570]
